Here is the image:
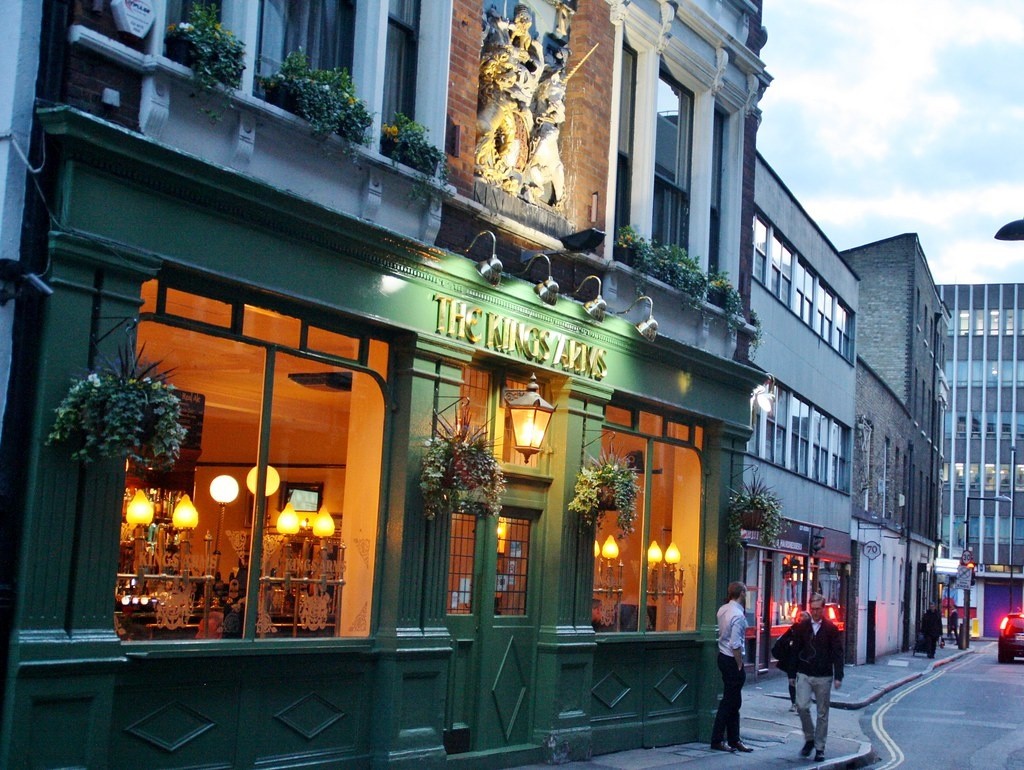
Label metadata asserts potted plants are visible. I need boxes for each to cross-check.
[43,336,188,475]
[724,470,784,552]
[782,556,800,582]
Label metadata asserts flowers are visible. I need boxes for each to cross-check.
[616,226,730,293]
[380,112,430,143]
[419,404,507,522]
[259,45,376,168]
[568,442,643,541]
[166,0,237,48]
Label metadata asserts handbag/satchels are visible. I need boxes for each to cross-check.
[939,637,946,649]
[771,626,794,660]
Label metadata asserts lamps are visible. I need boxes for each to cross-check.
[665,540,680,600]
[125,488,155,575]
[210,474,240,555]
[312,505,337,580]
[172,494,199,575]
[499,365,558,464]
[454,230,503,286]
[288,372,353,393]
[0,273,55,307]
[564,275,608,323]
[512,253,559,306]
[602,534,620,598]
[276,502,301,578]
[757,372,776,412]
[647,540,662,600]
[246,348,281,496]
[616,296,658,342]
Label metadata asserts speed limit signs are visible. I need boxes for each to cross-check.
[961,550,974,564]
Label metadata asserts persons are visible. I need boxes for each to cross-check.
[922,602,943,659]
[710,581,754,753]
[771,592,844,762]
[947,604,960,645]
[474,4,566,206]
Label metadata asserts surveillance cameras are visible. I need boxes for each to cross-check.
[15,273,53,297]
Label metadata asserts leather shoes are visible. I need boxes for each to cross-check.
[728,740,754,751]
[711,742,733,751]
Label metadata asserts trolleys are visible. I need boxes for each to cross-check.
[912,629,930,657]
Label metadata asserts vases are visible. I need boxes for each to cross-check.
[380,138,439,176]
[265,83,365,144]
[164,35,247,91]
[589,482,619,511]
[614,245,724,306]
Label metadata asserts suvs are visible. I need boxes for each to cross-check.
[997,612,1024,664]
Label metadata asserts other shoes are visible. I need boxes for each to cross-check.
[790,704,796,711]
[802,740,814,756]
[815,749,825,762]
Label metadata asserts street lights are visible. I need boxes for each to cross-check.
[963,495,1013,649]
